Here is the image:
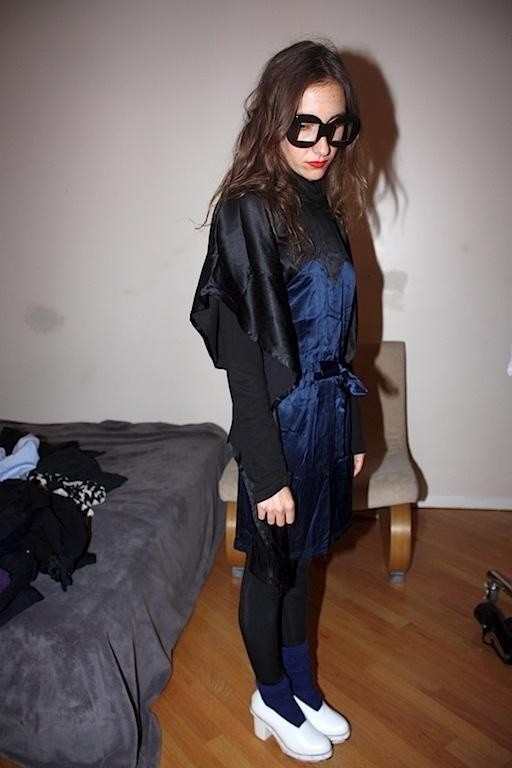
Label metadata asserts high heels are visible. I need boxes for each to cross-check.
[294,692,351,746]
[250,687,335,764]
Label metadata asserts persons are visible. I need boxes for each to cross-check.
[187,37,371,762]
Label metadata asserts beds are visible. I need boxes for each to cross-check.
[0,419,229,768]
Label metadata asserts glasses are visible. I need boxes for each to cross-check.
[285,112,362,148]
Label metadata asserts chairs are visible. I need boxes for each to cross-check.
[218,340,420,584]
